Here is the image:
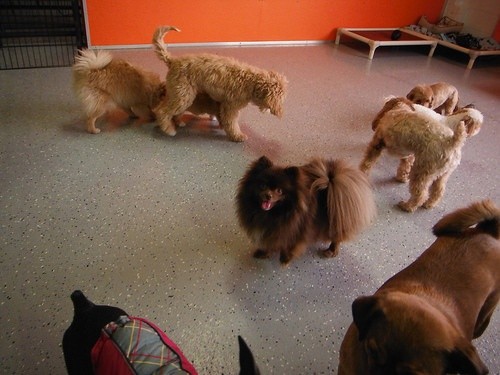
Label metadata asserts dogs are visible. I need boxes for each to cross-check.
[58,23,499,375]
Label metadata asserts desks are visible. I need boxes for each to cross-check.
[400,26,500,69]
[335,27,439,60]
